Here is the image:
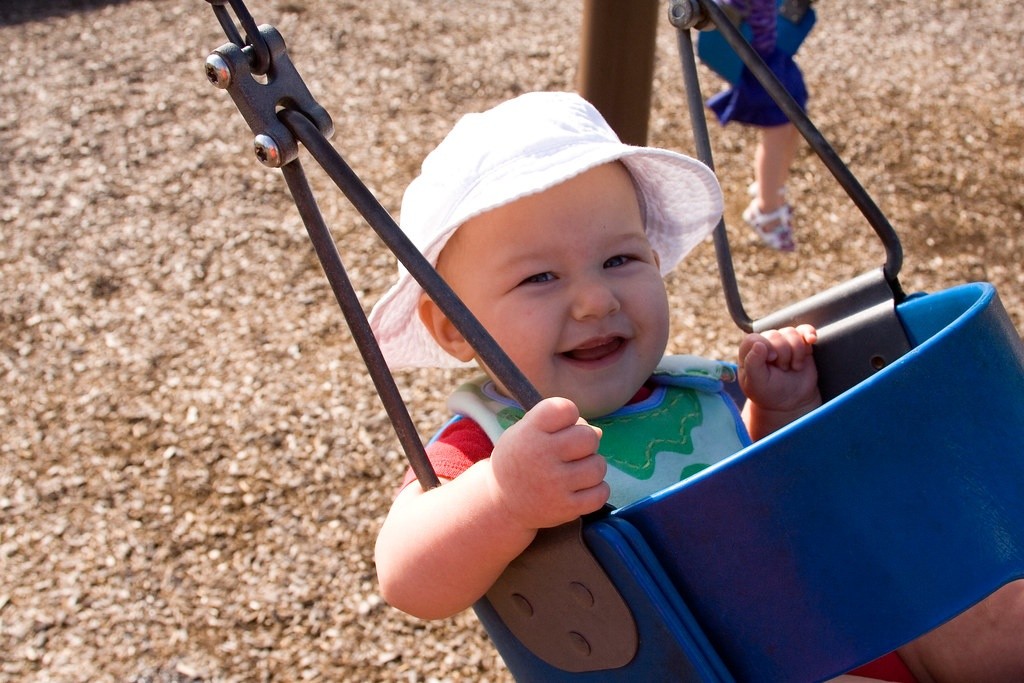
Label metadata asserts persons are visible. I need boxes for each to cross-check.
[366,91,1024,683]
[696,0,817,252]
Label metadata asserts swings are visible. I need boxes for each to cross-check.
[200,0,1024,683]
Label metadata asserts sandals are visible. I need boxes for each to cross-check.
[742,180,799,253]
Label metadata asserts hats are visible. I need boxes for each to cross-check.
[367,88,725,369]
